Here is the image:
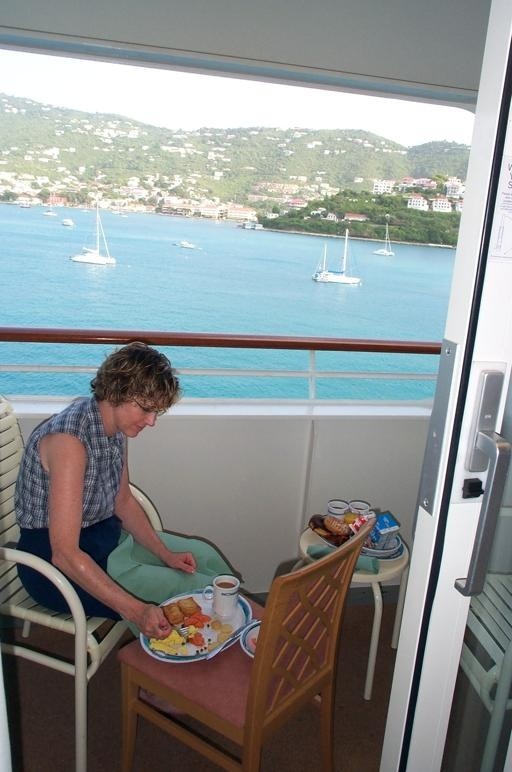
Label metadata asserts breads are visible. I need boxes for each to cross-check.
[161,597,202,625]
[207,619,233,652]
[310,510,359,537]
[249,637,257,649]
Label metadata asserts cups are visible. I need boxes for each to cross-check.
[202,574,239,616]
[329,500,372,523]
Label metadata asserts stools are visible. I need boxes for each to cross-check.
[290,527,410,701]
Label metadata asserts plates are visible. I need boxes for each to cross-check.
[316,516,405,561]
[140,589,252,664]
[240,620,260,657]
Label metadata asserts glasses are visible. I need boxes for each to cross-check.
[134,399,167,416]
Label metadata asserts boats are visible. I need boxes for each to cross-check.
[63,219,73,226]
[172,239,196,250]
[41,209,58,218]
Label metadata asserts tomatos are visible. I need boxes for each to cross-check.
[183,613,210,645]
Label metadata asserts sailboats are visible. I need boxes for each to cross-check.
[311,228,361,285]
[70,205,117,265]
[372,221,395,257]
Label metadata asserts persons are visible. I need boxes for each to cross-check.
[13,340,265,715]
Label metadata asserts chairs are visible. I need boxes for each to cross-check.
[1,393,165,772]
[460,573,512,772]
[114,517,379,772]
